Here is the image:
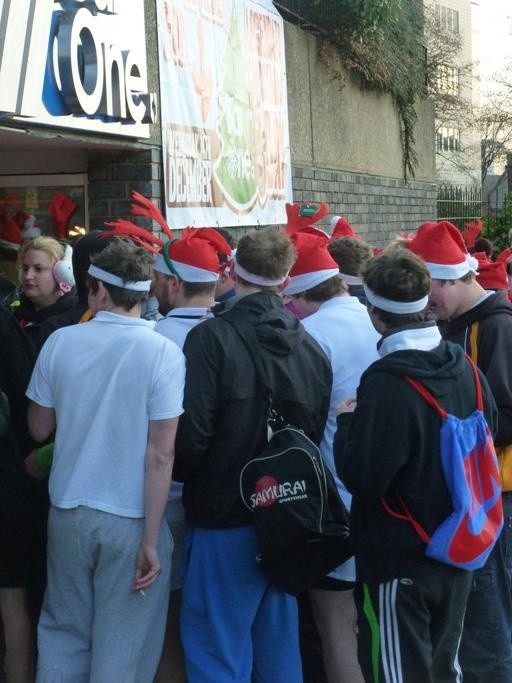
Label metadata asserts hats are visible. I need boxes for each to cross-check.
[0,211,41,253]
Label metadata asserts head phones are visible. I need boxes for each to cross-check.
[53,242,76,296]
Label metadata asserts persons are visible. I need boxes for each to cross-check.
[2,212,512,683]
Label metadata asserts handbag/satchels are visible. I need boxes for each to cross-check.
[239,423,352,587]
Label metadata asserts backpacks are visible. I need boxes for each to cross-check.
[380,352,505,573]
